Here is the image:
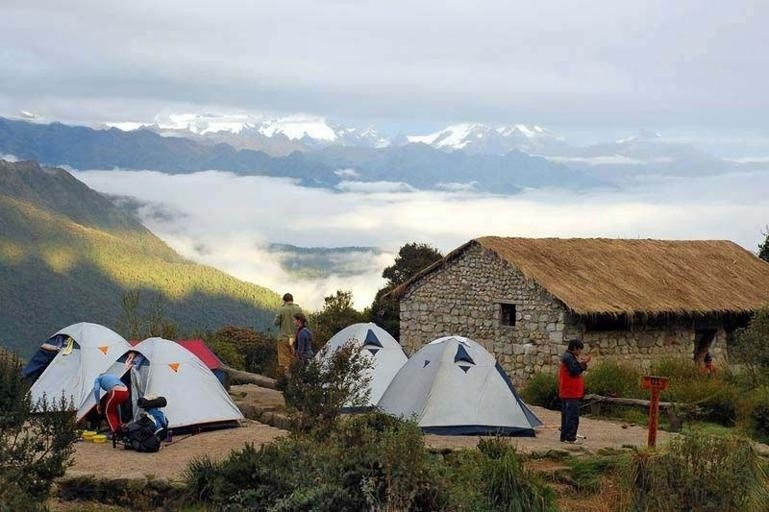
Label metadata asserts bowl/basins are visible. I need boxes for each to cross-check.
[83,431,97,442]
[92,435,107,443]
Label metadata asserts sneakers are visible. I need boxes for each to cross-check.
[564,439,582,444]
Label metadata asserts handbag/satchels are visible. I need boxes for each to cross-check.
[119,409,168,452]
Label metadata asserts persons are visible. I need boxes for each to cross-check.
[557,338,592,446]
[700,351,719,377]
[289,312,314,367]
[91,372,130,441]
[270,292,308,381]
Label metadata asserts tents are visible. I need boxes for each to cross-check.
[373,335,543,437]
[17,321,133,416]
[305,321,409,413]
[67,335,245,440]
